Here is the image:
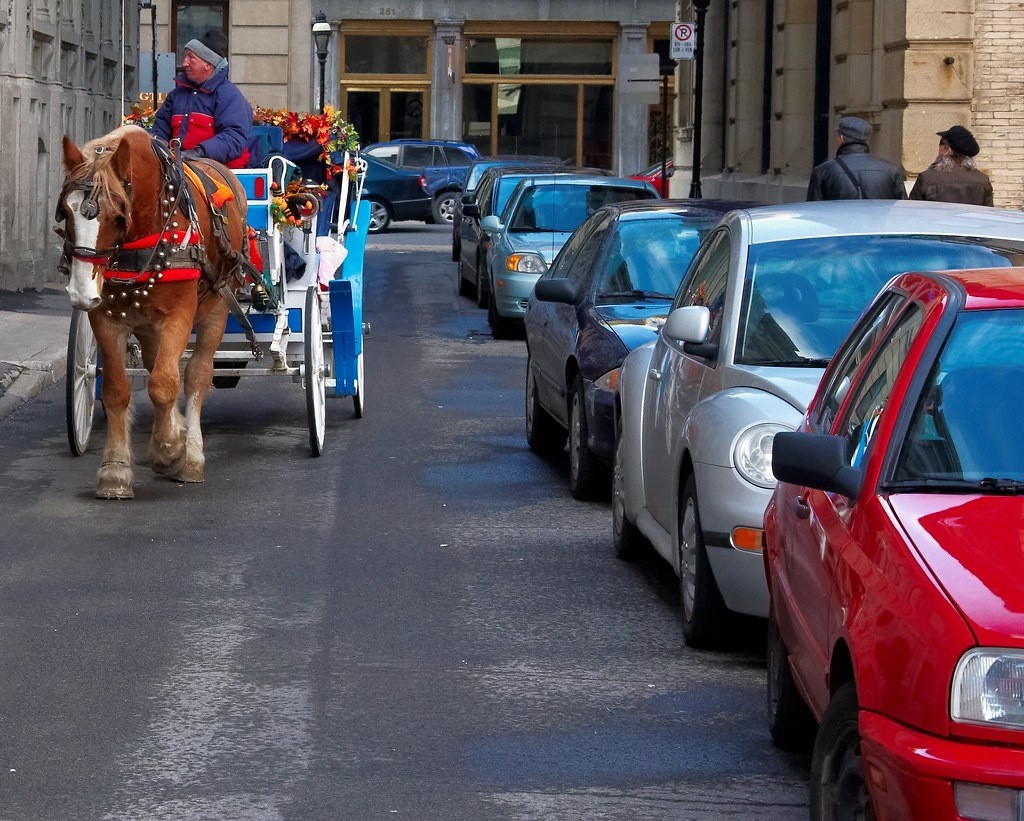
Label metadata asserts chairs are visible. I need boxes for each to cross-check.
[918,364,1024,472]
[738,271,838,360]
[521,203,564,227]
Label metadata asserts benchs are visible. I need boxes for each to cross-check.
[137,120,336,255]
[229,156,287,236]
[249,126,320,190]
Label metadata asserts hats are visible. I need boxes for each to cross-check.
[936,124,980,159]
[837,116,872,141]
[185,30,229,68]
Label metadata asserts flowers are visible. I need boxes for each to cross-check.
[116,101,360,183]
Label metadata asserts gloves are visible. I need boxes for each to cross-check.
[179,144,205,156]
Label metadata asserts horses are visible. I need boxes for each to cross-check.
[54,123,250,500]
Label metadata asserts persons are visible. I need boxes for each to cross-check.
[150,30,253,170]
[806,117,909,202]
[908,125,993,208]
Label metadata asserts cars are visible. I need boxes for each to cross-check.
[517,198,780,504]
[626,156,675,199]
[331,151,435,236]
[762,262,1023,821]
[605,196,1024,653]
[451,155,610,309]
[478,169,666,341]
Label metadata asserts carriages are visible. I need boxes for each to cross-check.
[53,122,373,502]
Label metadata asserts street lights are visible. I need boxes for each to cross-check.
[309,9,332,116]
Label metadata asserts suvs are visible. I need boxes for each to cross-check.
[361,138,486,225]
